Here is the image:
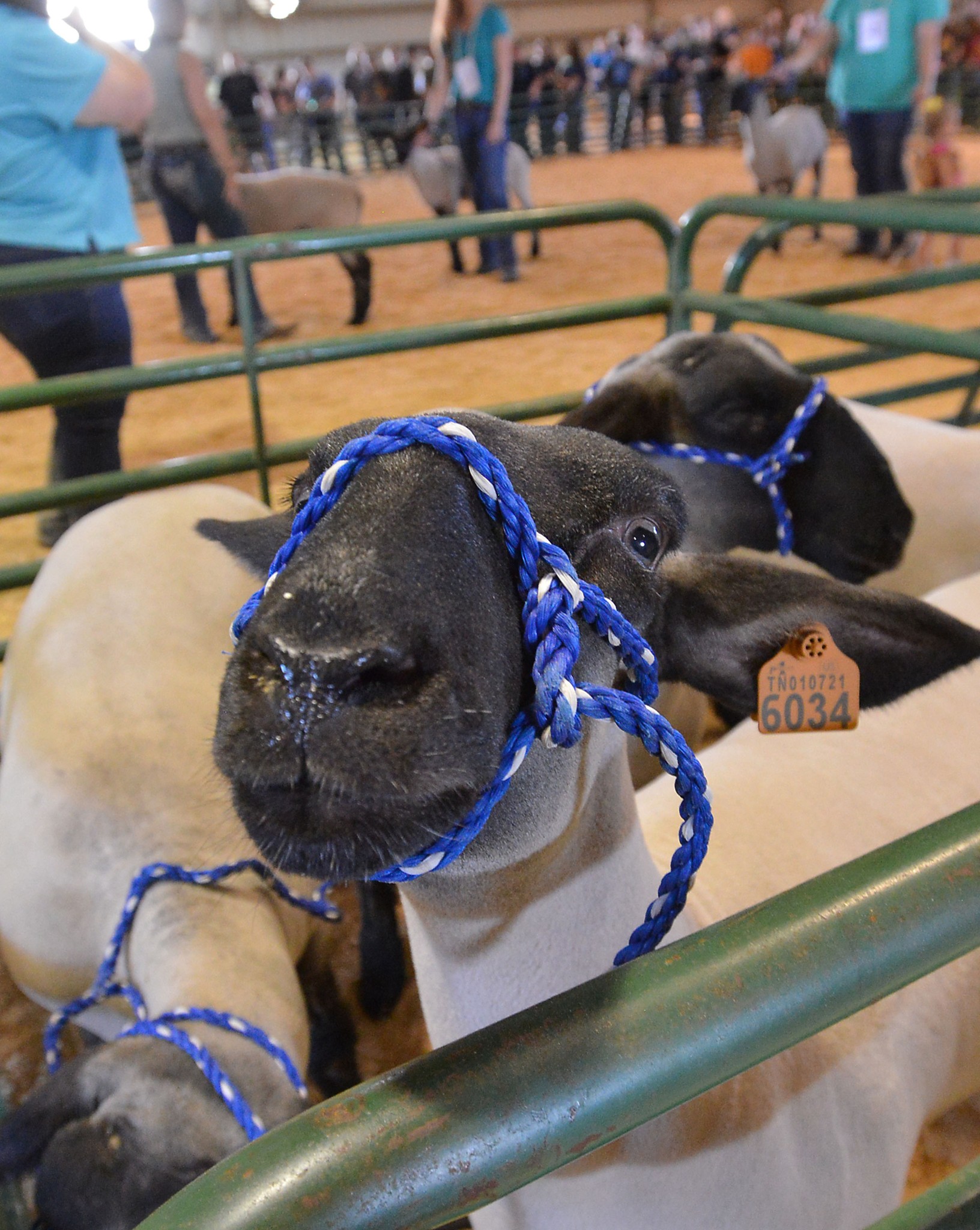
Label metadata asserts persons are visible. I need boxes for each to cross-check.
[776,0,949,263]
[938,6,980,129]
[0,0,156,550]
[215,42,435,175]
[505,8,829,162]
[139,0,273,347]
[425,0,519,283]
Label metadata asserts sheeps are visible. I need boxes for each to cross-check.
[2,326,977,1228]
[233,168,375,327]
[725,79,828,250]
[413,143,543,275]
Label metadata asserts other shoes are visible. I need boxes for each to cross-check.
[37,519,68,548]
[254,318,302,342]
[183,322,218,342]
[882,243,899,260]
[503,267,518,281]
[476,260,500,272]
[844,243,875,254]
